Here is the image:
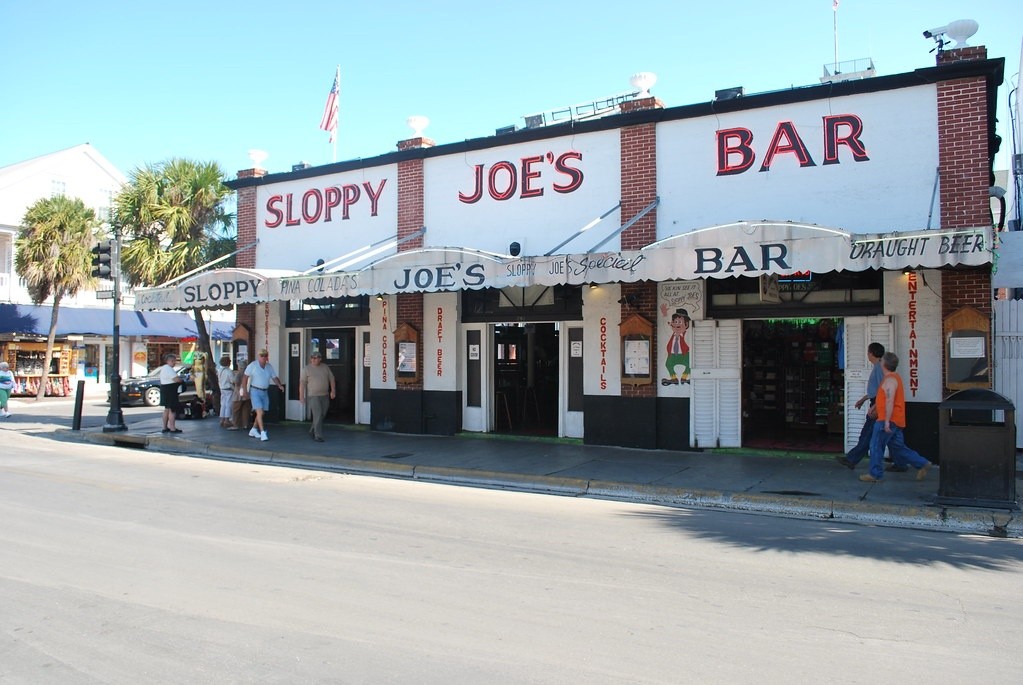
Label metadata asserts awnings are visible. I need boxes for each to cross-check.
[133,217,993,312]
[203,314,237,339]
[0,305,200,338]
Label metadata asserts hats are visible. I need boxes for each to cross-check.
[311,351,321,356]
[258,349,268,354]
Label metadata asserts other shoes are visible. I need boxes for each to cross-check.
[860,474,883,482]
[224,422,233,425]
[836,455,854,470]
[227,426,238,430]
[5,413,11,418]
[162,428,170,433]
[916,461,932,480]
[885,465,908,472]
[171,429,182,433]
[220,421,224,425]
[314,438,324,443]
[309,431,315,438]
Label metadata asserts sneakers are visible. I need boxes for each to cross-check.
[248,428,261,438]
[260,431,268,441]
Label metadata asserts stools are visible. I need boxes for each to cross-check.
[495,386,513,431]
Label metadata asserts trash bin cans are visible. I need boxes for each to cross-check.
[935,387,1018,508]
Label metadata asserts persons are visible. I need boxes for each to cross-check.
[299,351,336,442]
[0,362,17,418]
[243,348,284,441]
[227,361,252,430]
[161,354,183,434]
[860,352,932,482]
[218,357,236,427]
[836,342,908,472]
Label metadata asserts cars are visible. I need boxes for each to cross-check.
[107,363,194,407]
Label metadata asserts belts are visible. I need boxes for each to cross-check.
[250,385,268,391]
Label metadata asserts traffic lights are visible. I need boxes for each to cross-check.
[97,240,117,280]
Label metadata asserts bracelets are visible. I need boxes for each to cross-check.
[885,415,891,418]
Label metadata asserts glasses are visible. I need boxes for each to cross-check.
[259,354,268,357]
[311,356,320,359]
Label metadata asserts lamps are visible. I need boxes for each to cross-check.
[316,259,325,273]
[589,282,598,289]
[901,268,912,275]
[510,241,520,257]
[376,295,383,300]
